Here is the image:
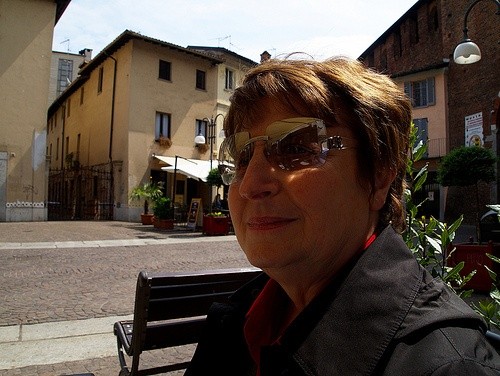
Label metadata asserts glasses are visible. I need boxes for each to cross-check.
[216,116,374,185]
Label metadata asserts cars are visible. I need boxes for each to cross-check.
[476,209,500,258]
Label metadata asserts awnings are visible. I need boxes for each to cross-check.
[156,156,234,182]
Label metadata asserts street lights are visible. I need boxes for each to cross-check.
[194,112,226,215]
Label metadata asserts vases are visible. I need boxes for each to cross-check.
[202,214,229,235]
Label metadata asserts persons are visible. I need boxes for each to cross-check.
[184,52,500,376]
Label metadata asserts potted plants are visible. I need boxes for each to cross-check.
[436,144,500,296]
[153,196,176,229]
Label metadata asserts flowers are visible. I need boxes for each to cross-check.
[207,211,225,216]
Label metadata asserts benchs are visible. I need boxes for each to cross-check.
[112,267,262,376]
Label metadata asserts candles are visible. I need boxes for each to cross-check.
[131,176,165,224]
